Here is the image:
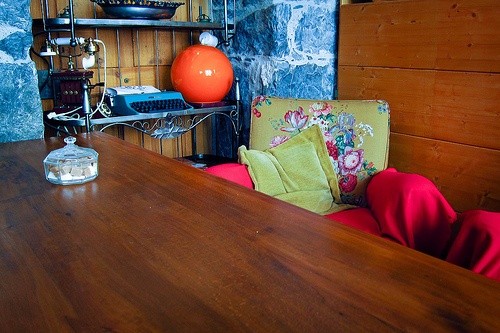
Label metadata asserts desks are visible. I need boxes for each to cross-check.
[0,131,500,333]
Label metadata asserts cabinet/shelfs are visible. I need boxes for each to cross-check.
[31,0,242,169]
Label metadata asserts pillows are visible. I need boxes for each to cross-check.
[237,123,359,216]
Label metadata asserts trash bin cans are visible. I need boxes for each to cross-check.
[185,154,222,167]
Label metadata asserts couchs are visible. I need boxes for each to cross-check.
[203,95,457,248]
[441,210,500,282]
